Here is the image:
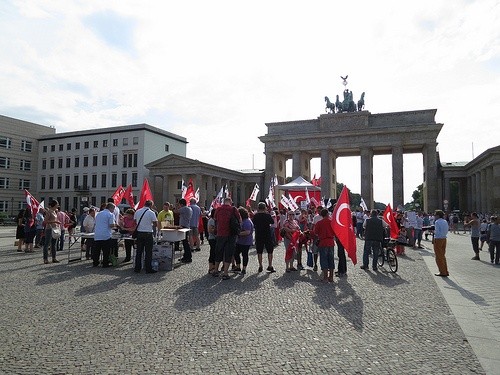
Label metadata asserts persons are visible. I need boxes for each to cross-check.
[121,202,179,262]
[360,209,388,270]
[431,210,449,277]
[350,207,424,255]
[415,210,461,248]
[276,199,347,282]
[16,200,77,252]
[133,200,158,273]
[461,212,500,264]
[207,197,277,279]
[43,200,61,264]
[172,198,208,263]
[80,198,119,267]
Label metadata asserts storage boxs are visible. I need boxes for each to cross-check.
[160,227,192,242]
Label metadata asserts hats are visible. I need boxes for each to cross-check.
[83,207,90,212]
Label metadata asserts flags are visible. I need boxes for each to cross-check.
[383,204,399,239]
[25,191,39,228]
[182,179,199,205]
[111,180,153,209]
[331,186,358,265]
[360,197,367,211]
[210,176,322,213]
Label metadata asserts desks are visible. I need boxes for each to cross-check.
[66,234,185,272]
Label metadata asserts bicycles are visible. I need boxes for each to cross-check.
[377,236,399,273]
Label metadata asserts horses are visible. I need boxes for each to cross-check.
[325,89,365,113]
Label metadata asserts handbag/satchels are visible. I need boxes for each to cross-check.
[51,226,62,238]
[132,229,137,239]
[307,252,314,267]
